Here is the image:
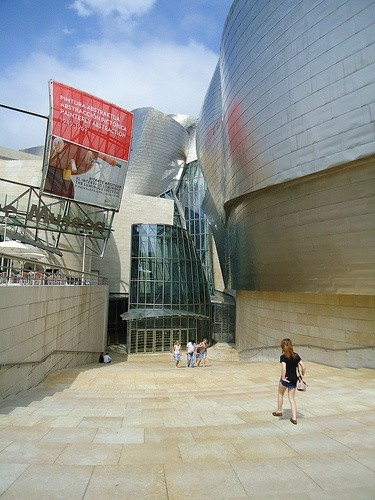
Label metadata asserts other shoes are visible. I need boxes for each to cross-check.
[272,411,281,416]
[290,418,298,423]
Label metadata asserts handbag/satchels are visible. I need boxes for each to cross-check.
[297,377,308,390]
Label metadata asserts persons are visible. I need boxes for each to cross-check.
[173,341,182,368]
[99,352,112,363]
[186,338,208,368]
[272,339,306,424]
